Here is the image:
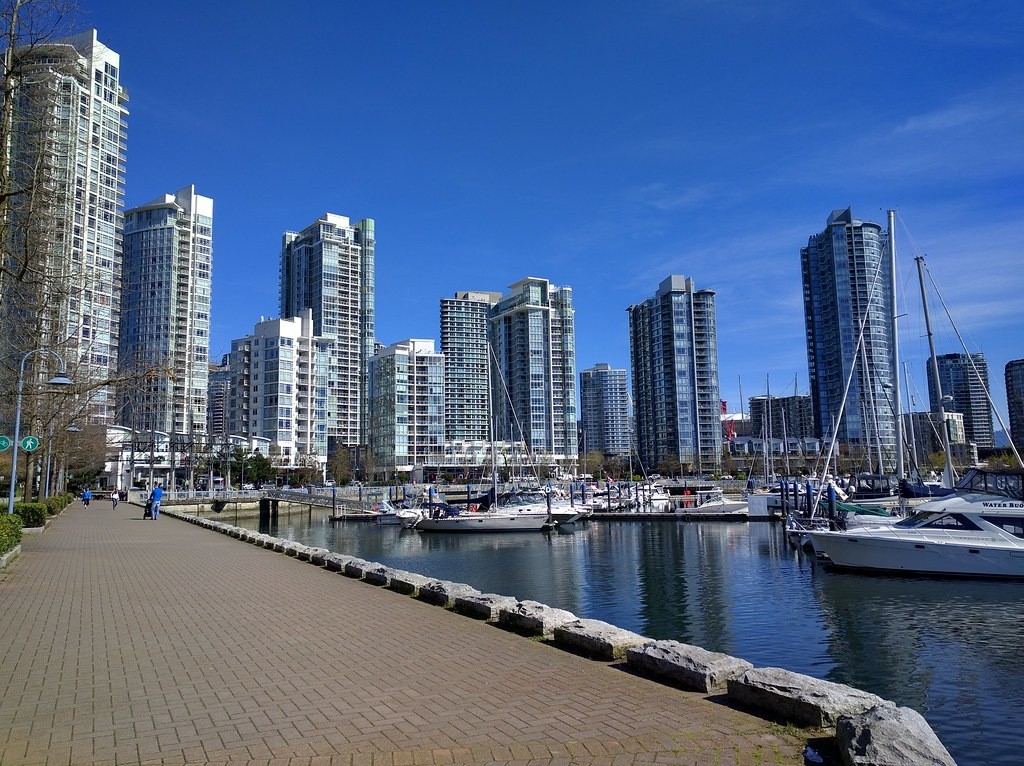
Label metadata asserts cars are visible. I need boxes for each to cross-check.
[648,474,661,479]
[260,482,277,489]
[243,483,254,490]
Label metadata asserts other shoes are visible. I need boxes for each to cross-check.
[85,504,86,509]
[113,506,115,510]
[151,518,153,520]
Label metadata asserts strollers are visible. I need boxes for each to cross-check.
[143,499,153,520]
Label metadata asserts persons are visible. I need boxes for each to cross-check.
[149,481,163,520]
[110,487,119,510]
[82,487,91,509]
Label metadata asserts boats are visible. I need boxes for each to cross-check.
[328,501,400,525]
[397,508,444,529]
[676,497,748,513]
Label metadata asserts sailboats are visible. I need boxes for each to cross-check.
[413,344,594,532]
[743,205,1024,576]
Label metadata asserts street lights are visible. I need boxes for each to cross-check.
[44,410,81,498]
[241,461,251,489]
[8,348,75,514]
